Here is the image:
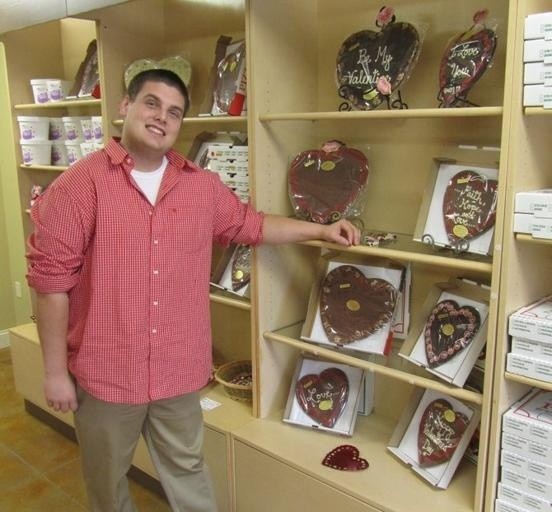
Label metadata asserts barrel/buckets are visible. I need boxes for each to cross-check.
[17,115,107,167]
[31,78,65,104]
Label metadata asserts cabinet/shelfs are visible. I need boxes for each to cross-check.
[2,2,99,427]
[102,0,258,512]
[483,2,550,512]
[229,1,512,512]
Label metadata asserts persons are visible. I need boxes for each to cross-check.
[26,68,360,512]
